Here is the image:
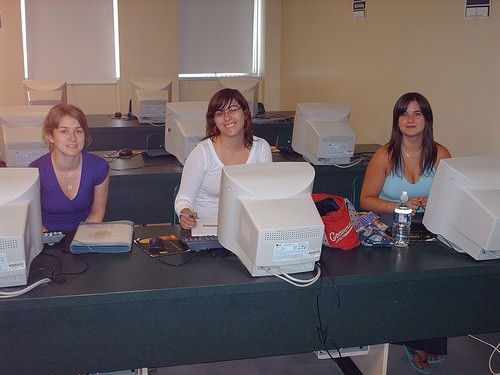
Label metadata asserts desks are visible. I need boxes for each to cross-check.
[83,143,381,224]
[2,212,500,375]
[83,110,296,152]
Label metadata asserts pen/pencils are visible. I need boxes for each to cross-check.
[178,213,199,219]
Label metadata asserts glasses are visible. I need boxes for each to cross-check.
[213,106,242,117]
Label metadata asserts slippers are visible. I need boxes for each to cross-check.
[424,353,444,364]
[403,344,429,374]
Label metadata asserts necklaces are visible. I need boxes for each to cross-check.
[55,161,76,190]
[401,145,420,158]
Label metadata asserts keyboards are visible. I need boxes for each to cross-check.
[42,231,64,246]
[180,235,223,251]
[145,149,171,157]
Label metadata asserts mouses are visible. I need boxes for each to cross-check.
[115,112,121,117]
[119,150,132,156]
[148,237,165,251]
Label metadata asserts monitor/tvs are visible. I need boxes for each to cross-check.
[219,78,259,118]
[0,105,54,168]
[129,79,172,124]
[216,161,324,279]
[0,166,44,288]
[423,155,500,260]
[22,79,67,107]
[291,102,355,166]
[164,101,211,165]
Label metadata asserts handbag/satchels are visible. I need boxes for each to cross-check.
[311,194,361,251]
[359,224,396,248]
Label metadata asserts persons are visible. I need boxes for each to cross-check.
[28,104,110,233]
[360,92,451,374]
[174,88,273,229]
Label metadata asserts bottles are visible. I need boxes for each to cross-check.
[392,191,412,247]
